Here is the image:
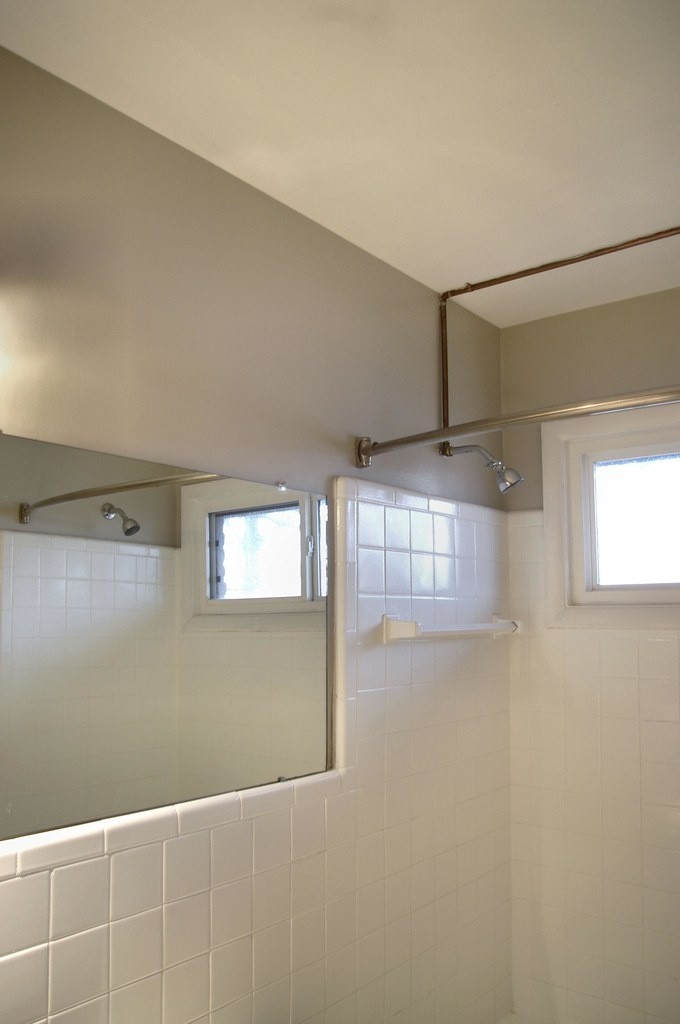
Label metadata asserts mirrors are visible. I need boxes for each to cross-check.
[0,432,328,842]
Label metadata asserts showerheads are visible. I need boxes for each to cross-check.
[487,459,522,494]
[122,515,140,537]
